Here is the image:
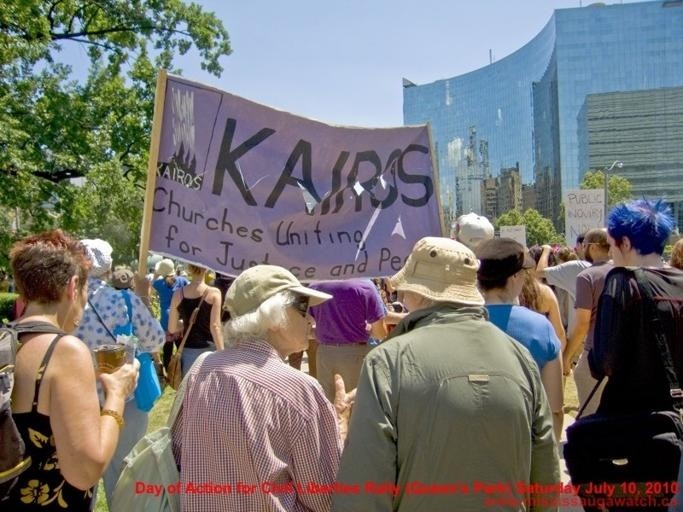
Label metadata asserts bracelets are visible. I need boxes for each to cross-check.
[100,410,124,432]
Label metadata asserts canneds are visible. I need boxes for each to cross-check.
[93,343,126,401]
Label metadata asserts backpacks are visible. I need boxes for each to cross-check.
[1,318,60,484]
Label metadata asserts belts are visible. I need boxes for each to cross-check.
[320,342,367,346]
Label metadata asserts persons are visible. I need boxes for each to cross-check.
[331,236,561,512]
[71,237,166,511]
[582,194,683,512]
[166,263,342,512]
[0,228,140,512]
[110,211,617,443]
[667,239,682,270]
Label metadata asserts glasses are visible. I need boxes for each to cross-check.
[574,246,580,251]
[582,242,600,248]
[291,296,309,318]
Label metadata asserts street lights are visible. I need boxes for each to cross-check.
[603,159,624,226]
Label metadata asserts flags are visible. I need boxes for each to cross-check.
[138,67,445,282]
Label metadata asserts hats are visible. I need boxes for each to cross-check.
[450,213,493,249]
[390,236,485,305]
[222,265,333,316]
[155,259,176,276]
[475,237,536,276]
[82,238,113,277]
[112,269,134,288]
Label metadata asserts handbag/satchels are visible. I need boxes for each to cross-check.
[563,266,683,511]
[168,353,181,391]
[109,350,213,512]
[114,289,162,411]
